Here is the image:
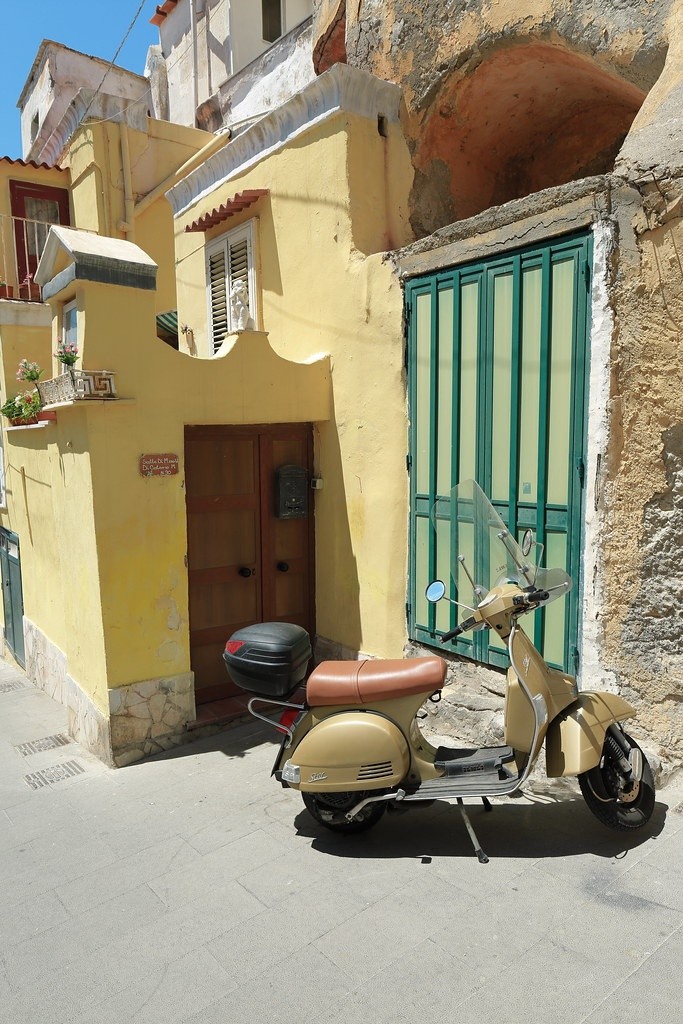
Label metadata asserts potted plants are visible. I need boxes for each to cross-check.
[0,276,13,300]
[1,396,38,426]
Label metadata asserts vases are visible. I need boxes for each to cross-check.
[36,411,57,422]
[185,334,193,349]
[19,283,41,302]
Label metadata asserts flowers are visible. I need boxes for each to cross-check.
[51,336,79,394]
[16,358,45,401]
[180,322,193,335]
[13,387,42,417]
[17,273,36,284]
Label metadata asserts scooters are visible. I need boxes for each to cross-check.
[220,476,657,864]
